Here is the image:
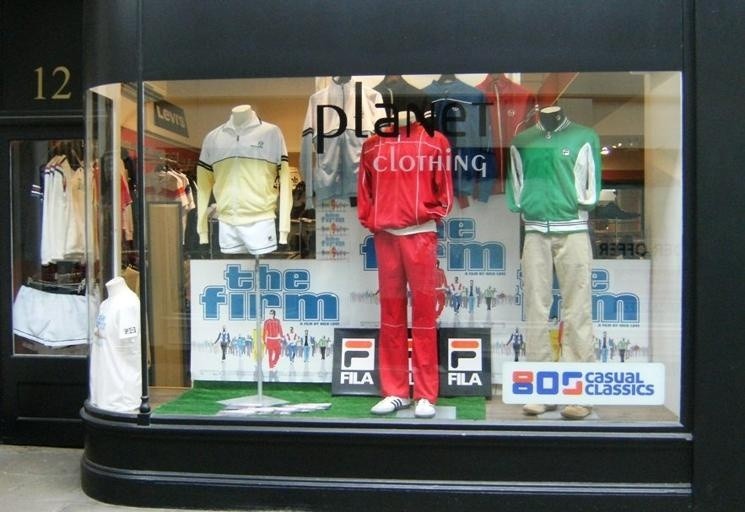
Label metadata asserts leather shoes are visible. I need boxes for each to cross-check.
[595,201,640,219]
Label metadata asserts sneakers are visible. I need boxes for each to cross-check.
[415,397,435,418]
[523,404,557,414]
[370,396,411,415]
[560,405,593,419]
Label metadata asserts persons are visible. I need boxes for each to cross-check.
[197,105,293,254]
[506,106,601,421]
[357,112,453,419]
[89,277,143,413]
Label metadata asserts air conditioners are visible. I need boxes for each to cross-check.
[121,79,168,105]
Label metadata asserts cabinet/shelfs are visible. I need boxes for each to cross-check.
[207,211,316,258]
[589,182,643,258]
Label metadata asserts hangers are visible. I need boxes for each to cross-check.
[49,139,81,166]
[26,272,86,294]
